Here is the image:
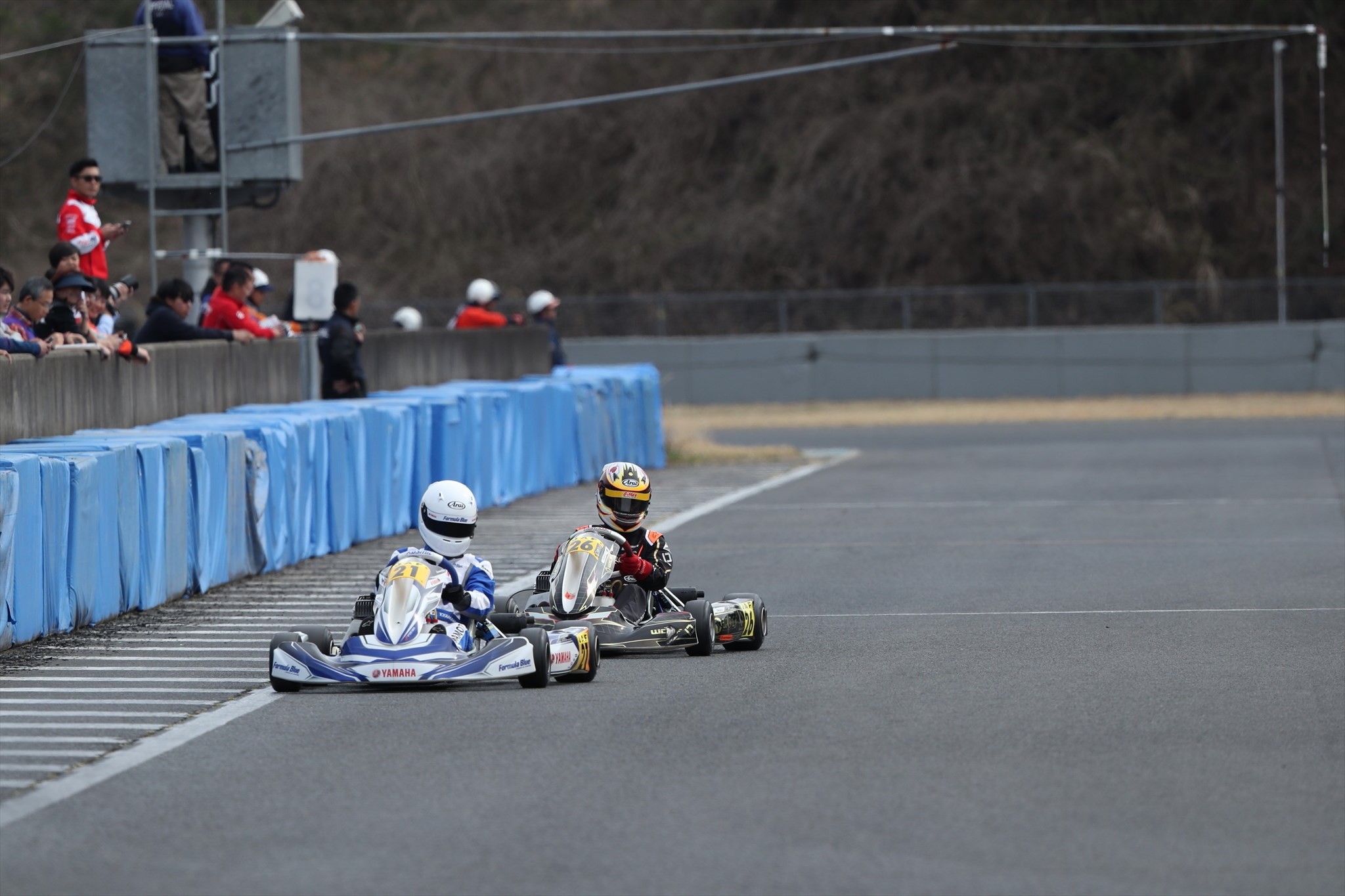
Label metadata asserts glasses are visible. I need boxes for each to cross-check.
[176,290,194,304]
[78,175,103,182]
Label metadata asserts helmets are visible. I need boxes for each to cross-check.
[417,480,479,557]
[392,306,423,332]
[527,290,561,314]
[465,278,500,304]
[595,462,652,533]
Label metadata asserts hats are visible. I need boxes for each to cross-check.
[253,268,274,292]
[55,273,93,289]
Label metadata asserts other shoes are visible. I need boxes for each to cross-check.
[428,624,447,635]
[358,617,374,635]
[537,601,550,607]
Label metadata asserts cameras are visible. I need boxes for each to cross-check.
[107,274,140,300]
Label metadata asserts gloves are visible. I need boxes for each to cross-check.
[619,552,652,580]
[442,582,465,606]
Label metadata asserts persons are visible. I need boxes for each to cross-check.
[447,278,566,365]
[283,249,340,331]
[56,156,127,280]
[136,0,219,174]
[550,462,673,625]
[358,480,495,655]
[0,241,301,366]
[391,306,423,331]
[318,282,367,399]
